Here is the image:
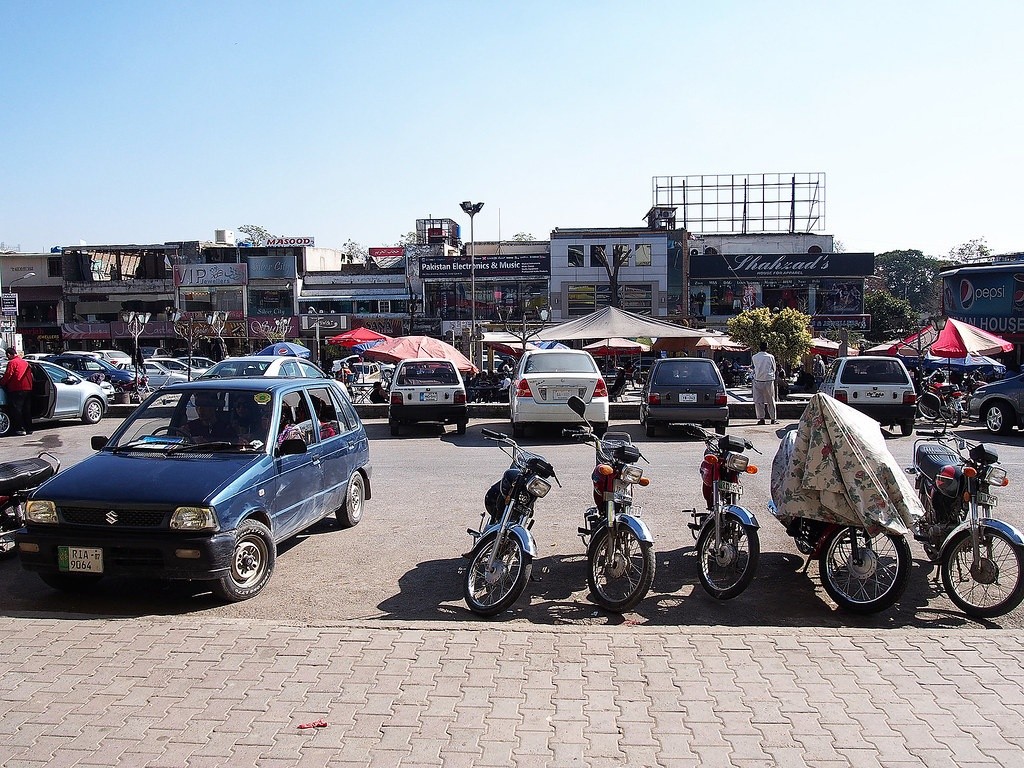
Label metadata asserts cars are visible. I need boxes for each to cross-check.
[15,376,372,601]
[818,356,917,437]
[0,346,237,438]
[968,372,1024,435]
[507,349,730,440]
[195,355,469,435]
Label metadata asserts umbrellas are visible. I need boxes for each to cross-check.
[257,342,312,358]
[650,326,750,362]
[326,326,480,385]
[478,305,730,387]
[864,313,1014,390]
[808,335,859,357]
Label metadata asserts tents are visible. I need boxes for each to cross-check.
[581,345,643,379]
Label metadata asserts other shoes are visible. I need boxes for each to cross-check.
[16,430,26,435]
[758,420,765,425]
[771,420,779,424]
[28,429,34,434]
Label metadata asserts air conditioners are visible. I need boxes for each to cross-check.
[662,210,673,218]
[690,247,703,256]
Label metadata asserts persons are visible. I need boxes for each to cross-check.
[0,347,33,436]
[1005,359,1018,378]
[466,360,513,403]
[100,375,116,393]
[751,342,779,425]
[347,359,358,382]
[337,362,351,381]
[691,290,706,314]
[609,368,624,401]
[777,354,826,395]
[840,284,862,308]
[369,382,389,404]
[176,393,336,448]
[722,287,734,306]
[715,357,742,384]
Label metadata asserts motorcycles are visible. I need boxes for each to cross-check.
[917,368,966,427]
[669,423,763,600]
[766,393,912,618]
[0,452,61,544]
[461,427,562,618]
[112,375,157,404]
[905,430,1024,620]
[563,395,656,613]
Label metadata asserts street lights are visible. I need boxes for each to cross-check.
[167,307,219,382]
[890,315,949,396]
[10,273,36,348]
[121,311,152,386]
[460,201,485,357]
[497,305,552,353]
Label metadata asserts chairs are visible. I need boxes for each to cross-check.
[866,367,872,381]
[843,367,854,378]
